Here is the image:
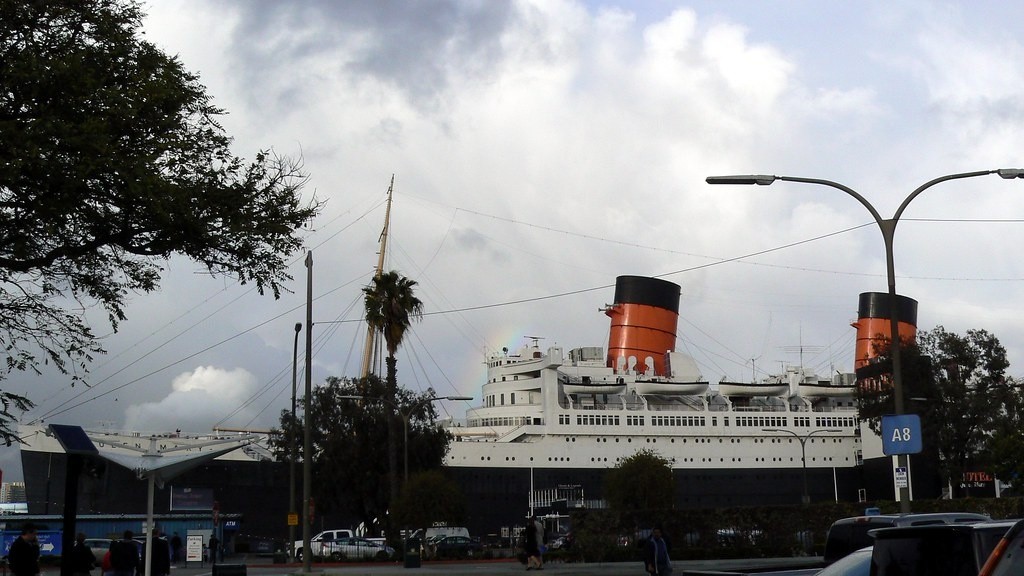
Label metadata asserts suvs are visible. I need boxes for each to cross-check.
[75,539,144,568]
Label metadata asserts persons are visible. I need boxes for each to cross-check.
[202,533,222,565]
[102,530,141,576]
[420,540,439,561]
[7,523,41,576]
[644,526,674,576]
[138,528,170,576]
[523,514,545,571]
[170,531,184,565]
[70,532,95,576]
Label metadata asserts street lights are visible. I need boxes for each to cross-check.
[761,428,842,556]
[705,168,1023,512]
[289,321,303,568]
[337,395,475,531]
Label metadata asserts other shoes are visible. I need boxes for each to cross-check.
[537,563,541,570]
[527,566,532,571]
[540,568,544,571]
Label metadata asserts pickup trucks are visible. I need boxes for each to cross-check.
[286,529,388,562]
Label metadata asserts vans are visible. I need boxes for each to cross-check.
[406,526,471,548]
[824,513,992,572]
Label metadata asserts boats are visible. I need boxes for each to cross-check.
[565,379,627,396]
[717,379,790,397]
[798,381,859,399]
[635,378,710,396]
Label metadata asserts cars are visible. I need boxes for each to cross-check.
[422,535,484,562]
[615,528,654,550]
[549,531,573,552]
[320,536,396,564]
[715,524,765,547]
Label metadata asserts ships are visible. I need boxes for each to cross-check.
[15,171,920,532]
